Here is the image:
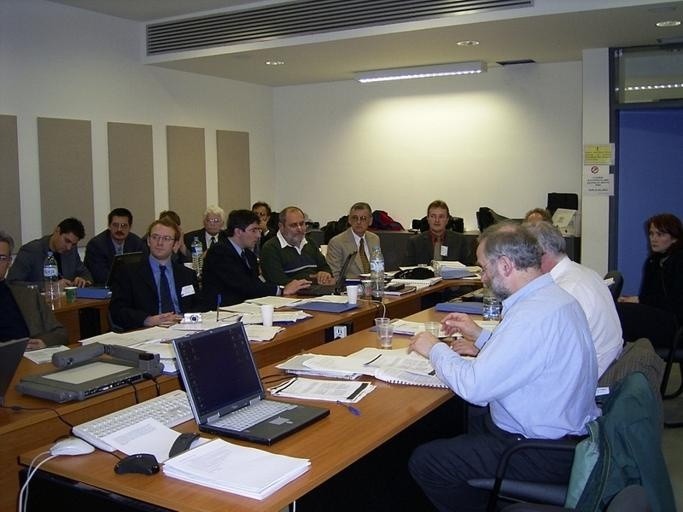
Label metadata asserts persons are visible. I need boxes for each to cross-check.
[615,214,683,349]
[6,202,278,339]
[261,207,336,286]
[0,229,67,351]
[406,200,473,310]
[326,203,382,282]
[451,221,625,381]
[202,210,312,309]
[408,220,603,512]
[525,208,553,224]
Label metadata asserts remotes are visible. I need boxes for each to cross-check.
[169,432,199,458]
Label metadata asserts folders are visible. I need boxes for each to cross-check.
[435,302,483,315]
[76,287,110,299]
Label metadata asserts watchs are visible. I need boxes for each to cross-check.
[279,285,285,296]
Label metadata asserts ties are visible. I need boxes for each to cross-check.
[433,237,443,260]
[359,238,370,273]
[159,266,176,314]
[241,251,248,263]
[210,237,215,245]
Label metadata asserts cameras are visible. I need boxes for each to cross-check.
[183,312,202,325]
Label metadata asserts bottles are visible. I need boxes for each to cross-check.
[43,251,60,312]
[483,283,500,320]
[369,246,385,298]
[191,237,203,280]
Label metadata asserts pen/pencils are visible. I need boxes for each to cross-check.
[337,400,360,416]
[217,294,221,321]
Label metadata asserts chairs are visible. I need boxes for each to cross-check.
[655,326,683,428]
[467,371,677,512]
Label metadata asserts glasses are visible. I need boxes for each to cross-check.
[206,217,223,223]
[255,212,269,216]
[480,255,505,275]
[350,216,371,222]
[150,234,176,242]
[113,222,128,230]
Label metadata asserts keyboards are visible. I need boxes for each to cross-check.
[71,389,195,453]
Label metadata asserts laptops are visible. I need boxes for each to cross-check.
[75,250,145,289]
[172,321,331,447]
[0,336,30,413]
[295,250,359,298]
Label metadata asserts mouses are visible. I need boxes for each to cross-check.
[47,436,95,457]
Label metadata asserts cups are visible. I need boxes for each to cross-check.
[346,285,358,304]
[260,305,274,327]
[361,280,373,297]
[320,245,328,258]
[425,322,442,339]
[345,278,361,292]
[378,324,393,349]
[360,274,371,280]
[374,318,390,340]
